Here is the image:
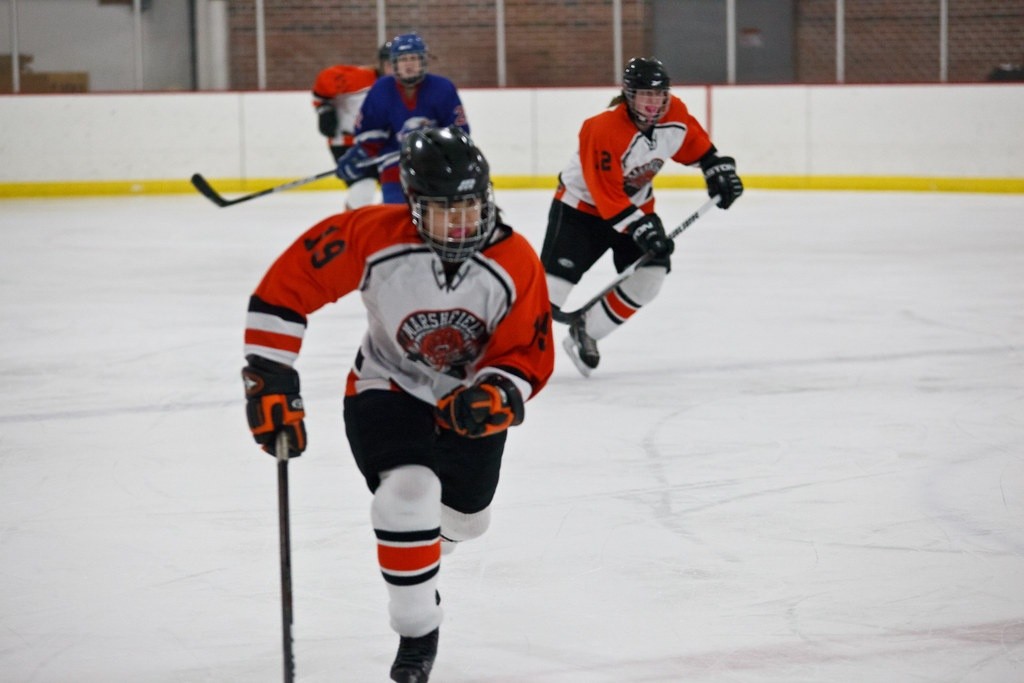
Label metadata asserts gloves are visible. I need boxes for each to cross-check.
[436,374,524,439]
[316,104,338,138]
[242,364,307,457]
[336,148,373,184]
[627,212,674,260]
[700,156,743,209]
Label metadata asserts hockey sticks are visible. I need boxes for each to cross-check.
[273,430,296,682]
[551,193,722,326]
[189,148,400,209]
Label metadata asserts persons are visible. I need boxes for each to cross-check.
[242,130,556,683]
[336,33,470,204]
[311,41,395,190]
[539,57,744,376]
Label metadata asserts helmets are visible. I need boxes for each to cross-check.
[391,33,426,87]
[400,129,495,264]
[377,42,396,76]
[622,57,670,131]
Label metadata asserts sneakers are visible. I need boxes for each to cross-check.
[389,627,439,683]
[562,314,599,378]
